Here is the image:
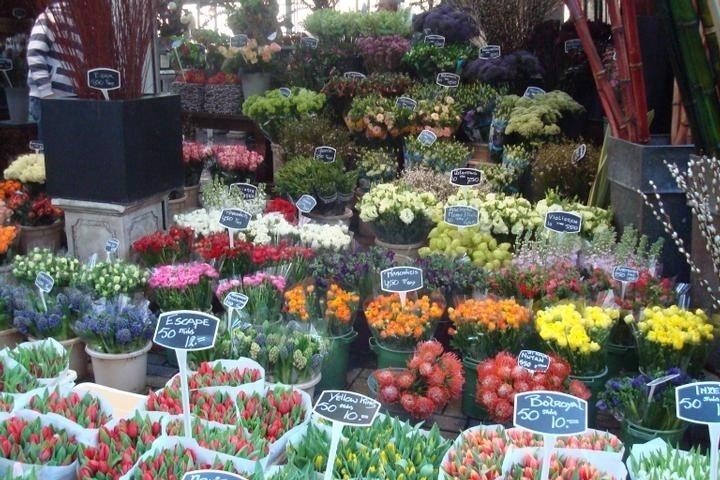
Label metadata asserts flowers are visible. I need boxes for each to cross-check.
[0,5,718,476]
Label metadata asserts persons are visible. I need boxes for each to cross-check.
[25,0,160,154]
[4,18,34,52]
